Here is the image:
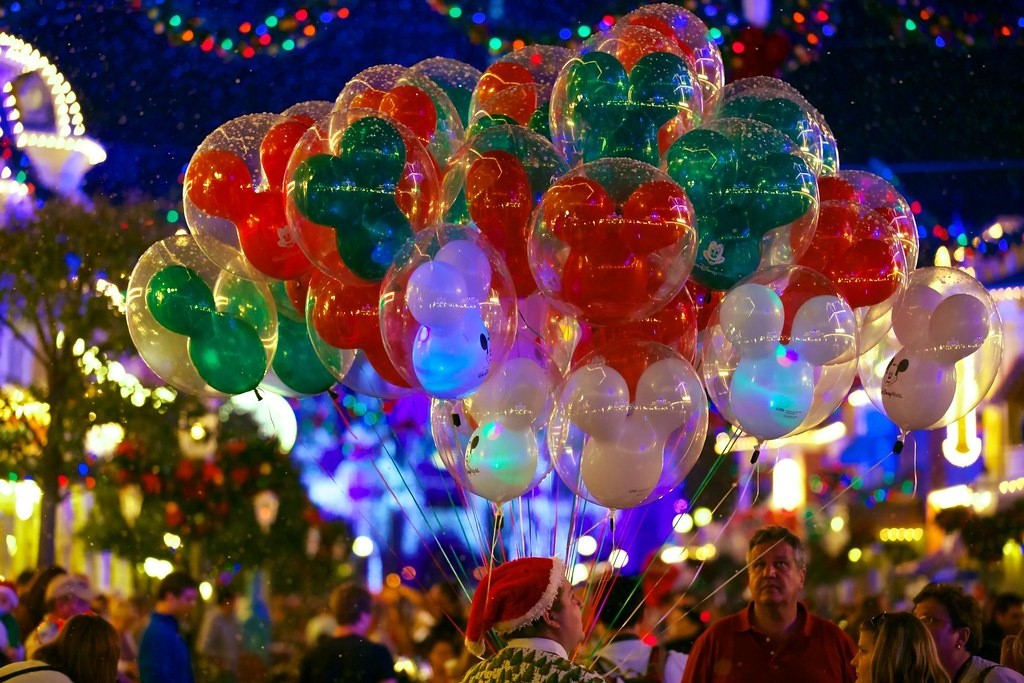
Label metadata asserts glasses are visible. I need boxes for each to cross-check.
[871,611,886,631]
[920,615,953,626]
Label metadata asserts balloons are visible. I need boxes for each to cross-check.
[125,4,1004,514]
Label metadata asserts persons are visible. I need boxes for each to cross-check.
[138,568,199,682]
[298,577,471,683]
[460,556,611,683]
[678,526,859,683]
[579,571,712,683]
[196,589,241,683]
[913,581,1024,683]
[0,562,138,683]
[850,611,954,683]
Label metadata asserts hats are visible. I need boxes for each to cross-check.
[44,574,95,602]
[465,556,565,661]
[1,581,18,607]
